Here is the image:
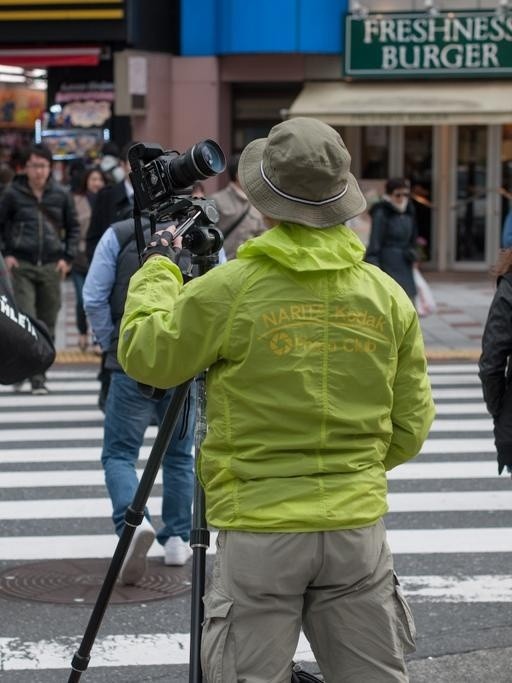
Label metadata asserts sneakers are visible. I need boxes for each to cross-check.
[31,380,48,395]
[163,536,194,567]
[117,517,156,586]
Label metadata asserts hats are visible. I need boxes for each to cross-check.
[235,116,368,230]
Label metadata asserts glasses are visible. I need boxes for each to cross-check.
[26,163,50,168]
[393,192,410,198]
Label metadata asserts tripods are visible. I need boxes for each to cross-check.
[68,200,224,683]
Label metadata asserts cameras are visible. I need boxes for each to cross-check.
[125,136,230,211]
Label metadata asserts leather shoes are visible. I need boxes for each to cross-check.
[79,336,89,351]
